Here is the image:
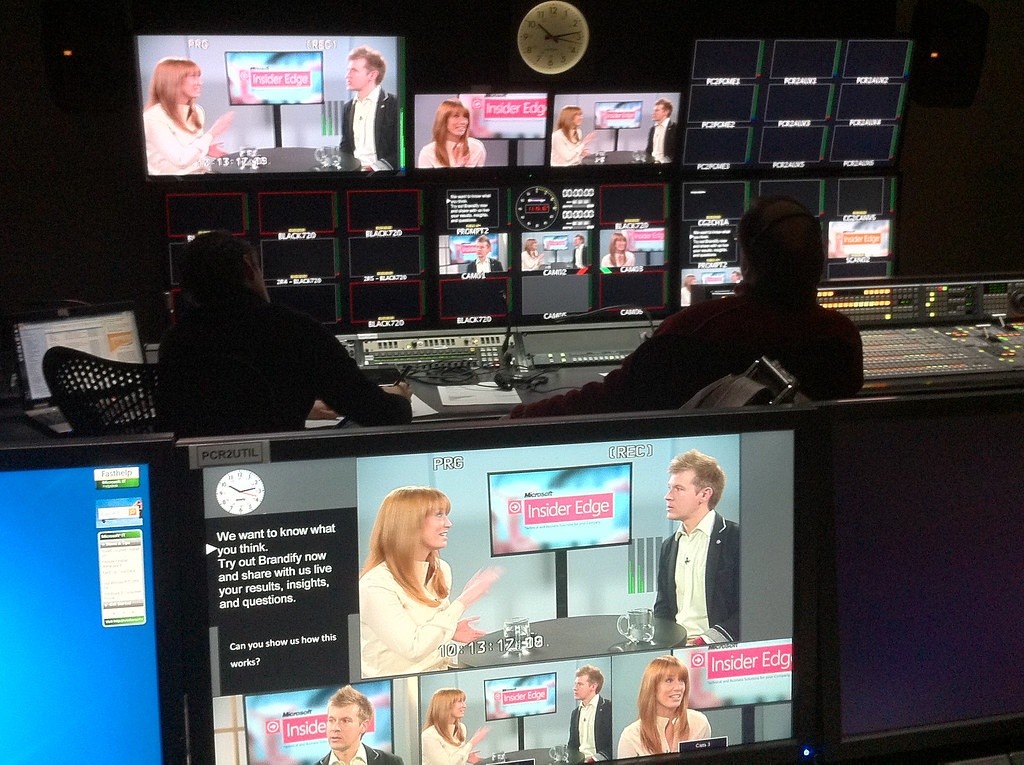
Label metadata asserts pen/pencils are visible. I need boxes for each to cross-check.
[392,365,413,387]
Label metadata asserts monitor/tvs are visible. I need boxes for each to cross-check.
[132,30,405,180]
[0,388,1024,764]
[411,90,682,168]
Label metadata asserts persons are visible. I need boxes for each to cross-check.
[420,689,488,765]
[567,665,612,763]
[418,99,486,168]
[732,271,743,284]
[572,234,588,269]
[653,450,740,647]
[142,59,232,176]
[358,487,499,679]
[338,48,398,172]
[496,197,866,419]
[157,232,411,436]
[681,274,696,307]
[600,233,635,268]
[618,655,712,759]
[521,239,545,271]
[466,234,503,273]
[316,685,404,765]
[646,99,678,165]
[550,105,597,166]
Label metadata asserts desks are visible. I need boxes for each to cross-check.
[473,748,585,765]
[457,616,686,669]
[210,149,361,172]
[581,151,654,165]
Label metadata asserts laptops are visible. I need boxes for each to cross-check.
[10,299,158,434]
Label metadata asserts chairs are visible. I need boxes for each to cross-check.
[41,346,228,437]
